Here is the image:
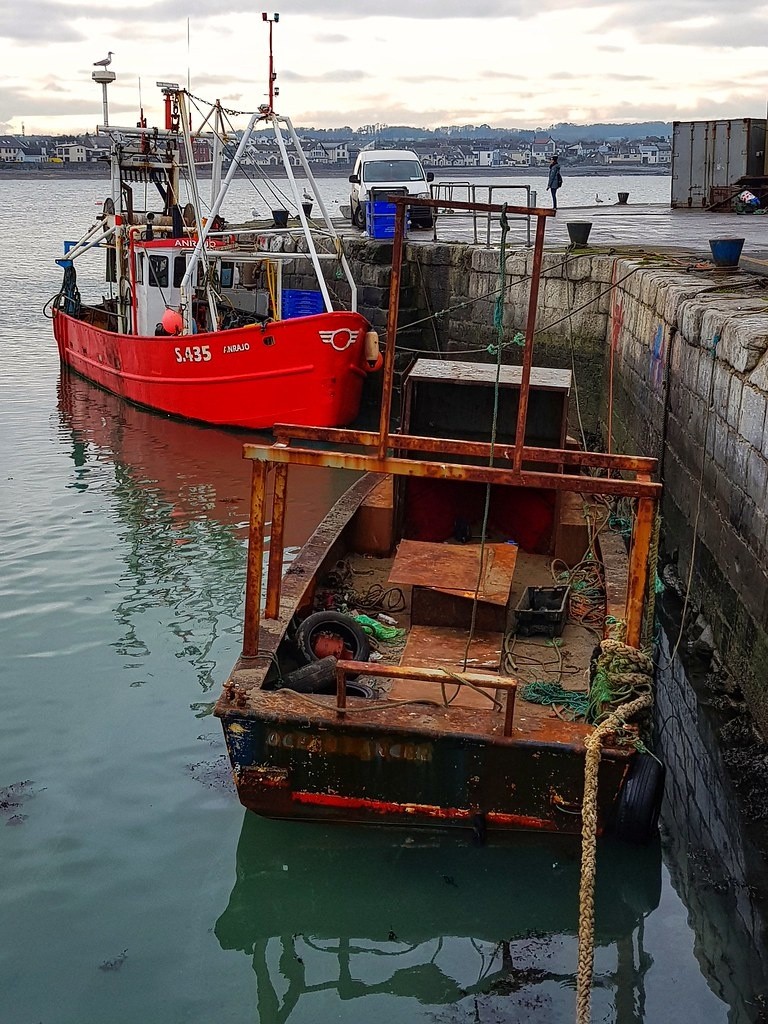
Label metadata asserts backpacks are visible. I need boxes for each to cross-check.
[557,173,563,188]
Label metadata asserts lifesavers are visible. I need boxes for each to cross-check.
[262,609,380,700]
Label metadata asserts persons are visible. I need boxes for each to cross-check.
[546,155,563,210]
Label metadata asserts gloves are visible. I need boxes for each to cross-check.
[546,186,550,191]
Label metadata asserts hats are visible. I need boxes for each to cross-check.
[550,155,558,160]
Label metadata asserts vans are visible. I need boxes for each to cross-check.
[349,149,437,230]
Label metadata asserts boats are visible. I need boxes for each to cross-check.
[43,12,666,845]
